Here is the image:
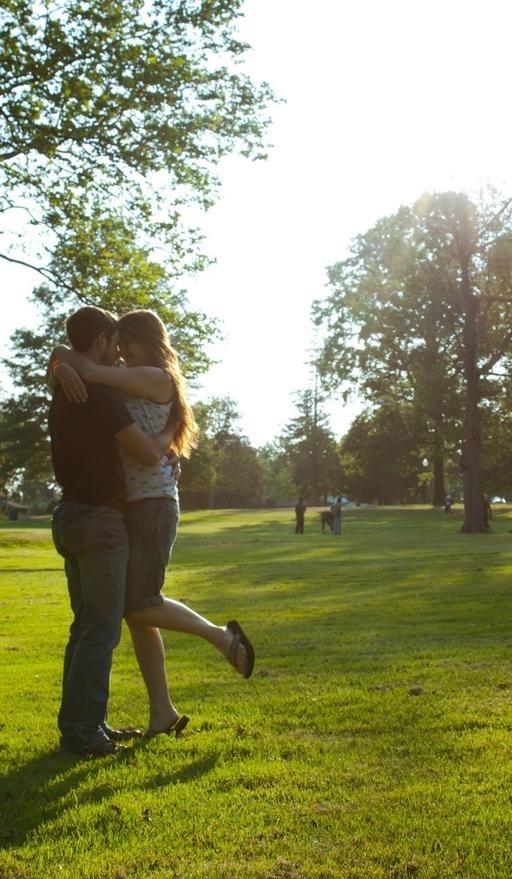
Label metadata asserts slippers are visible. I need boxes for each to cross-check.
[144,715,190,740]
[228,620,255,679]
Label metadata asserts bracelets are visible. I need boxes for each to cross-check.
[51,362,59,381]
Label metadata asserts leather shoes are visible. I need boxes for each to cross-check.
[108,728,142,740]
[69,739,136,757]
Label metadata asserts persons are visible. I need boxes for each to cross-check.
[331,496,342,535]
[443,492,454,515]
[50,306,182,760]
[321,510,334,534]
[481,494,493,533]
[295,496,306,534]
[46,309,255,746]
